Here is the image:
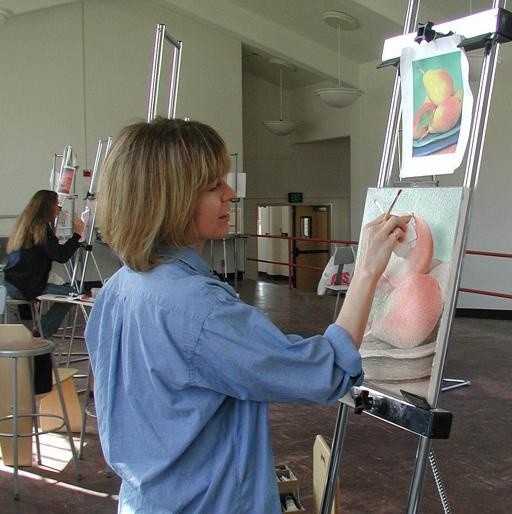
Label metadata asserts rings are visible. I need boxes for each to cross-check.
[391,230,399,240]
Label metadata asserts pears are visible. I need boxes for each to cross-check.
[412,66,461,143]
[363,200,449,350]
[373,275,392,293]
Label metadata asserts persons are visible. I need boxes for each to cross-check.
[79,115,416,512]
[2,187,86,342]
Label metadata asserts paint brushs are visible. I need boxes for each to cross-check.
[383,188,403,222]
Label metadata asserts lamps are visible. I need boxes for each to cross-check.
[314,11,365,108]
[263,58,299,135]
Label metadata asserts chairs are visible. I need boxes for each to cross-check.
[0,287,83,500]
